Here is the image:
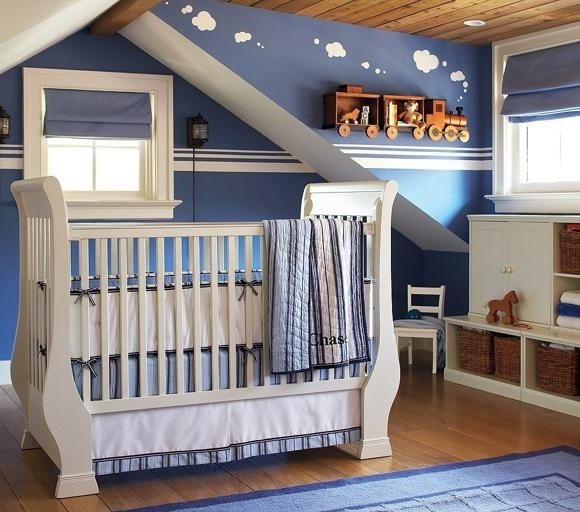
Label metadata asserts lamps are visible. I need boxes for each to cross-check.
[186,111,211,145]
[0,103,12,143]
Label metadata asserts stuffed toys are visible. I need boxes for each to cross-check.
[399,101,426,129]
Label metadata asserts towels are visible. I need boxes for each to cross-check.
[261,216,373,376]
[555,292,579,329]
[393,316,446,347]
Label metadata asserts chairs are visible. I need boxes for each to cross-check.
[394,283,448,377]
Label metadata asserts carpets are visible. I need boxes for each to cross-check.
[121,434,580,512]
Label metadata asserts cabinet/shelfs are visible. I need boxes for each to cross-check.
[550,212,580,335]
[442,313,524,402]
[522,322,579,418]
[466,212,558,335]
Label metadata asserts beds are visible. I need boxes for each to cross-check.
[8,175,403,500]
